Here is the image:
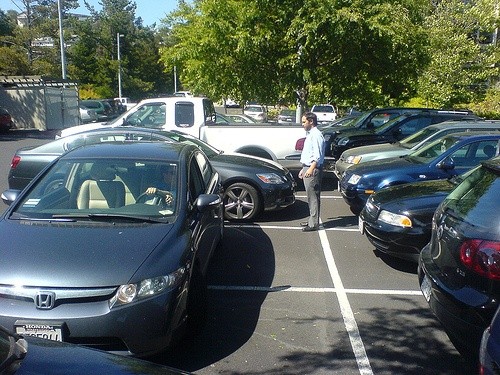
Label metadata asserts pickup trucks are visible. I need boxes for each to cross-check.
[54,97,314,172]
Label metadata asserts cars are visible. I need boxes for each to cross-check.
[77,98,137,122]
[244,104,268,124]
[276,109,296,126]
[0,140,226,357]
[358,163,487,267]
[0,324,201,375]
[221,94,247,108]
[337,134,500,210]
[212,114,257,125]
[0,127,297,223]
[479,300,500,375]
[321,106,500,183]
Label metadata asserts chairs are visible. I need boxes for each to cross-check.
[77,163,125,210]
[483,145,496,158]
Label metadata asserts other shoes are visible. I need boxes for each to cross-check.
[300,221,308,226]
[302,226,318,232]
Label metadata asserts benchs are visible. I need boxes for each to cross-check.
[117,167,142,205]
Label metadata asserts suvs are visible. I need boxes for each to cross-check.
[418,156,500,350]
[311,104,337,127]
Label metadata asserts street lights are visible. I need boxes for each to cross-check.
[117,32,125,97]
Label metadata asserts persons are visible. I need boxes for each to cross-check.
[78,163,116,191]
[146,165,178,207]
[298,112,325,231]
